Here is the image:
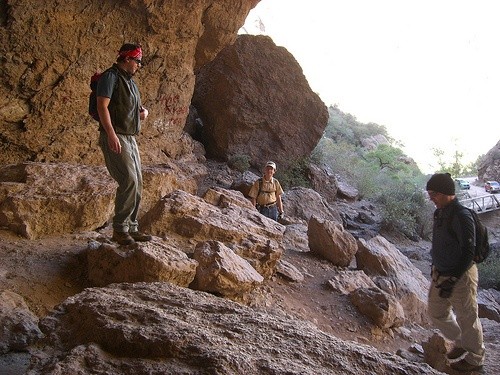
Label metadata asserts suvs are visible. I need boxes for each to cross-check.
[455,178,470,189]
[484,180,500,194]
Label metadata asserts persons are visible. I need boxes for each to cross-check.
[97,44,151,244]
[427,174,485,370]
[249,161,284,221]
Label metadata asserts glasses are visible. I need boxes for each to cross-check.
[132,58,141,64]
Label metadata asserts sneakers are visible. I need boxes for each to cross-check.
[113,232,136,245]
[130,231,151,241]
[450,359,482,371]
[448,347,466,359]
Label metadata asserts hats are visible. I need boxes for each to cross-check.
[265,161,276,171]
[426,173,455,195]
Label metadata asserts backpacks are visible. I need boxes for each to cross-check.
[447,205,489,264]
[89,70,119,122]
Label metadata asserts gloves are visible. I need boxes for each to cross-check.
[435,278,455,299]
